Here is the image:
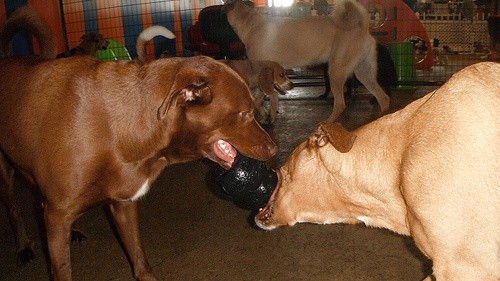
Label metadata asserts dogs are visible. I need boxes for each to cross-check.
[214,59,295,124]
[0,7,278,281]
[221,0,390,124]
[306,42,398,105]
[55,32,110,61]
[254,61,500,281]
[136,25,177,62]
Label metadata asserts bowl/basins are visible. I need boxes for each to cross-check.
[211,157,277,210]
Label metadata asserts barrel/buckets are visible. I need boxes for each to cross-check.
[388,41,418,89]
